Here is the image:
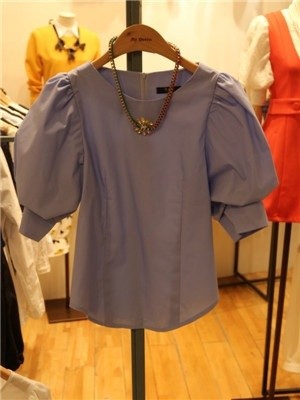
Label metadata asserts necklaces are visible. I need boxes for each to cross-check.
[53,24,85,61]
[108,35,181,135]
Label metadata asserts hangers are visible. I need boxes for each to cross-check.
[54,0,224,93]
[0,89,29,137]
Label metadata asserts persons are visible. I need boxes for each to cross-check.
[238,0,300,221]
[22,13,104,107]
